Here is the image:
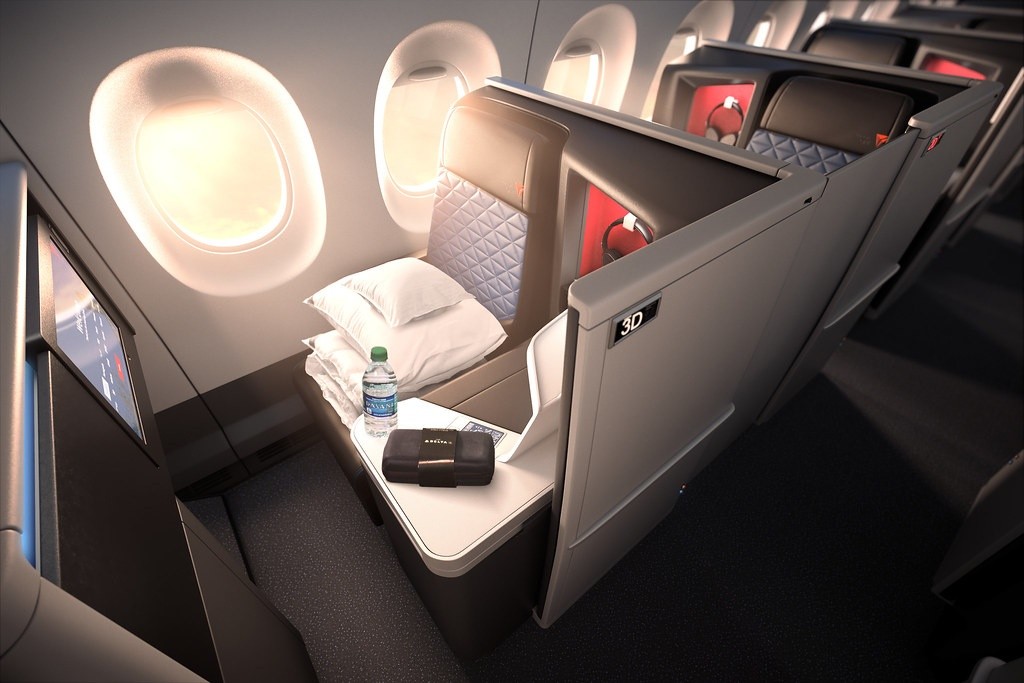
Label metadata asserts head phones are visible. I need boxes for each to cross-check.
[705,102,743,147]
[602,217,652,264]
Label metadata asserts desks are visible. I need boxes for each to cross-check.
[349,309,568,661]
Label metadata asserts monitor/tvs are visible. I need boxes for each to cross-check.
[24,214,159,465]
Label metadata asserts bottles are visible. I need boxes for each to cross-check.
[360,346,398,436]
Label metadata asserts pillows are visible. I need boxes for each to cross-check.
[304,352,359,430]
[303,280,510,383]
[301,329,368,412]
[346,254,476,327]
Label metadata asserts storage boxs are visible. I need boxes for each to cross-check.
[384,427,494,488]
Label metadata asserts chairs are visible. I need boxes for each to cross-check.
[809,24,911,66]
[747,77,919,175]
[301,86,572,530]
